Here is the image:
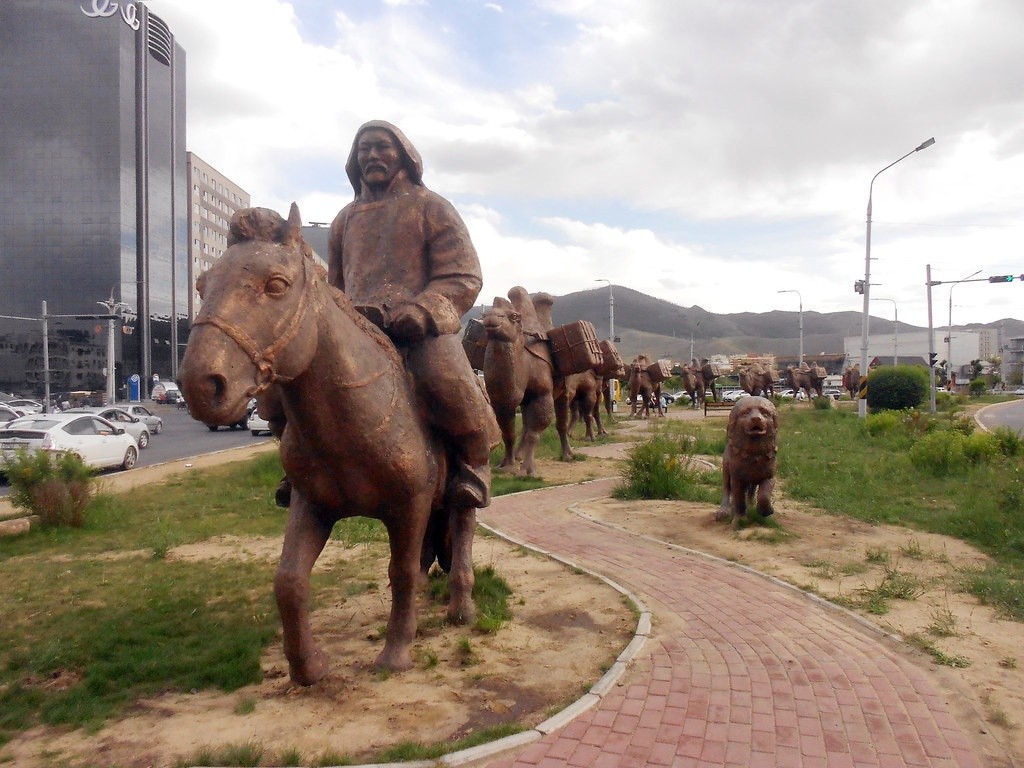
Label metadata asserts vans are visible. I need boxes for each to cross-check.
[1014,387,1024,394]
[938,388,947,392]
[812,390,841,400]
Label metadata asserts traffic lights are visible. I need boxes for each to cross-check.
[988,276,1013,284]
[76,315,119,320]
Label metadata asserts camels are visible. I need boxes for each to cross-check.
[785,362,827,402]
[841,364,860,402]
[460,285,625,481]
[622,353,672,421]
[680,357,722,410]
[737,363,780,399]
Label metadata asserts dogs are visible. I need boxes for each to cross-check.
[711,396,780,529]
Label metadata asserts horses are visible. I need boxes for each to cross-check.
[176,206,492,685]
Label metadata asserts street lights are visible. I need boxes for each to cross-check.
[595,280,614,343]
[858,137,936,418]
[947,270,982,393]
[777,290,803,368]
[870,299,897,366]
[691,321,700,362]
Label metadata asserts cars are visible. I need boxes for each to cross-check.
[728,392,751,397]
[673,391,693,401]
[626,395,645,405]
[779,390,803,399]
[62,407,151,448]
[705,392,712,397]
[247,409,278,436]
[0,405,20,429]
[0,392,20,402]
[2,399,45,417]
[113,405,163,434]
[723,391,733,397]
[660,393,674,404]
[0,414,140,476]
